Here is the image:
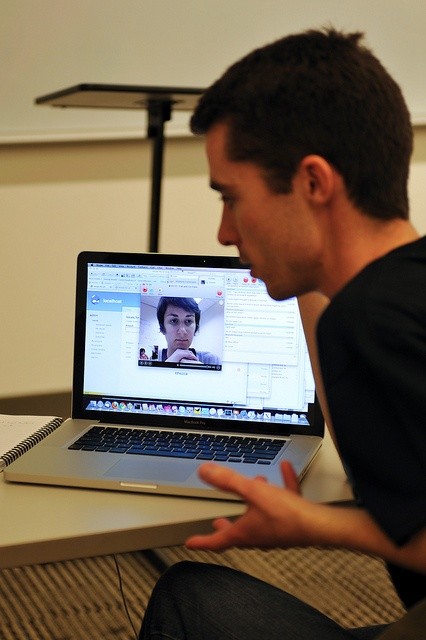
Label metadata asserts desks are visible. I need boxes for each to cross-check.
[0,394,356,568]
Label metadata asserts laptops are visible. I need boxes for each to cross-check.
[3,251,324,503]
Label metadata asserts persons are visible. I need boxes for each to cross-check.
[140,348,149,359]
[157,296,221,365]
[140,28,426,640]
